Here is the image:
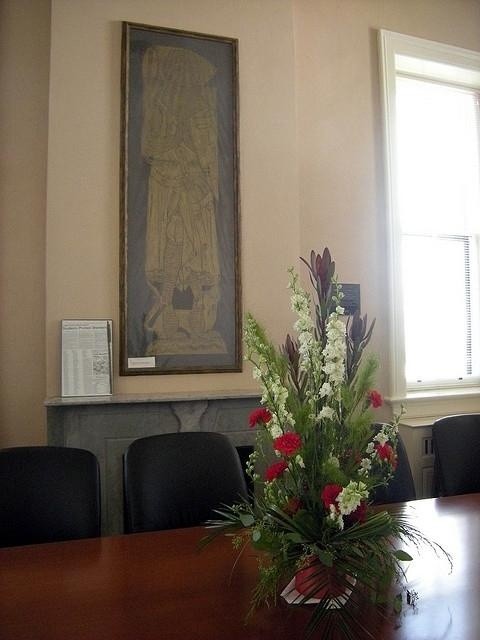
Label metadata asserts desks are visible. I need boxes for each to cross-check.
[0,492,480,640]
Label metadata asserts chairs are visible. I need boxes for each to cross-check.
[432,414,480,497]
[0,445,102,549]
[355,422,417,507]
[125,431,252,535]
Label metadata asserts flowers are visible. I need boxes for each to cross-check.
[195,247,455,640]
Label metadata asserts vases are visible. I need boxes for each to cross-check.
[295,555,346,599]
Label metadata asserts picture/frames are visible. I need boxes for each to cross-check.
[119,21,243,377]
